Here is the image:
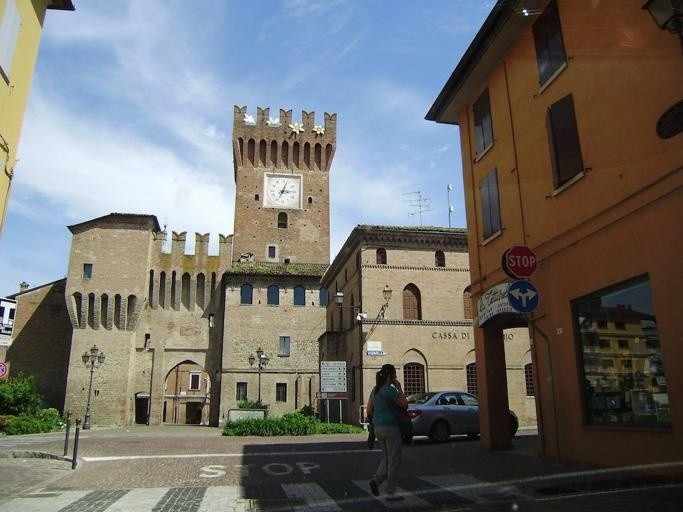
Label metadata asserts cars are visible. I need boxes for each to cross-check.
[403,391,519,443]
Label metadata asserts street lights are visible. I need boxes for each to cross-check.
[248,347,270,403]
[81,344,106,429]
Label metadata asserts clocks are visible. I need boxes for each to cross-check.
[261,172,303,210]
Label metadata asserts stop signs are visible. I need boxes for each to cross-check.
[501,245,538,279]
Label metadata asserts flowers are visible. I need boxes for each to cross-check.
[288,121,326,136]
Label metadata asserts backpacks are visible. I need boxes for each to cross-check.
[399,409,415,446]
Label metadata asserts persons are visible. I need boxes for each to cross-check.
[366,364,408,500]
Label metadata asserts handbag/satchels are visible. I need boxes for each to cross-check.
[367,424,375,450]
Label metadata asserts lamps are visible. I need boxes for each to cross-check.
[640,0,682,33]
[334,288,360,309]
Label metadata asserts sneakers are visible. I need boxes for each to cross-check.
[369,480,379,497]
[384,496,405,501]
